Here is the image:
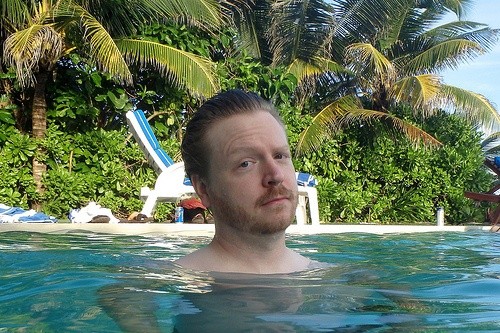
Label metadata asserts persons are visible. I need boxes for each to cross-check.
[172,90,313,273]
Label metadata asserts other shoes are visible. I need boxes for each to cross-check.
[191,213,204,224]
[205,214,215,224]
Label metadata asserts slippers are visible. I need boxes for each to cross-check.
[89,215,110,223]
[120,211,153,223]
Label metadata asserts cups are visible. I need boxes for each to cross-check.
[175,207,184,224]
[436,207,444,226]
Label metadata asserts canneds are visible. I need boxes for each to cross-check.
[174,207,184,224]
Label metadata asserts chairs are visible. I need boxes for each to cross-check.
[124,109,320,225]
